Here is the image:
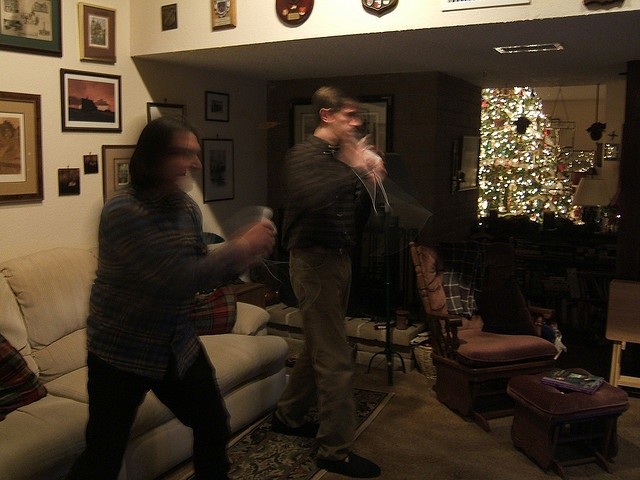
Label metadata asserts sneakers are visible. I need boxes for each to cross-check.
[317,452,381,478]
[272,415,319,438]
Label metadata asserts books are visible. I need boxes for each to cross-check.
[542,367,605,395]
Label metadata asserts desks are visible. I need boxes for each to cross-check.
[608,279,640,394]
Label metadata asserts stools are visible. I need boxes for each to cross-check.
[507,366,630,474]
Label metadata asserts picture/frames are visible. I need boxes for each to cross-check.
[458,136,481,192]
[207,90,230,123]
[204,139,235,202]
[76,3,116,63]
[291,93,391,158]
[85,156,98,174]
[0,0,62,55]
[147,102,185,133]
[57,168,81,194]
[62,68,123,132]
[0,91,44,204]
[162,4,178,30]
[102,144,142,204]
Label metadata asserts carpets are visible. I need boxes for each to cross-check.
[181,388,397,479]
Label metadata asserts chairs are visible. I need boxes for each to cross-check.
[408,239,557,430]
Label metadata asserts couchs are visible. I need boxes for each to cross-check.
[0,246,289,464]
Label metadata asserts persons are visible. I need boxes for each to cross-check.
[270,86,384,477]
[69,114,275,480]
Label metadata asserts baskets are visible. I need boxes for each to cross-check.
[414,344,437,376]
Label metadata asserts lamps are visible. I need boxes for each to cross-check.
[573,176,610,232]
